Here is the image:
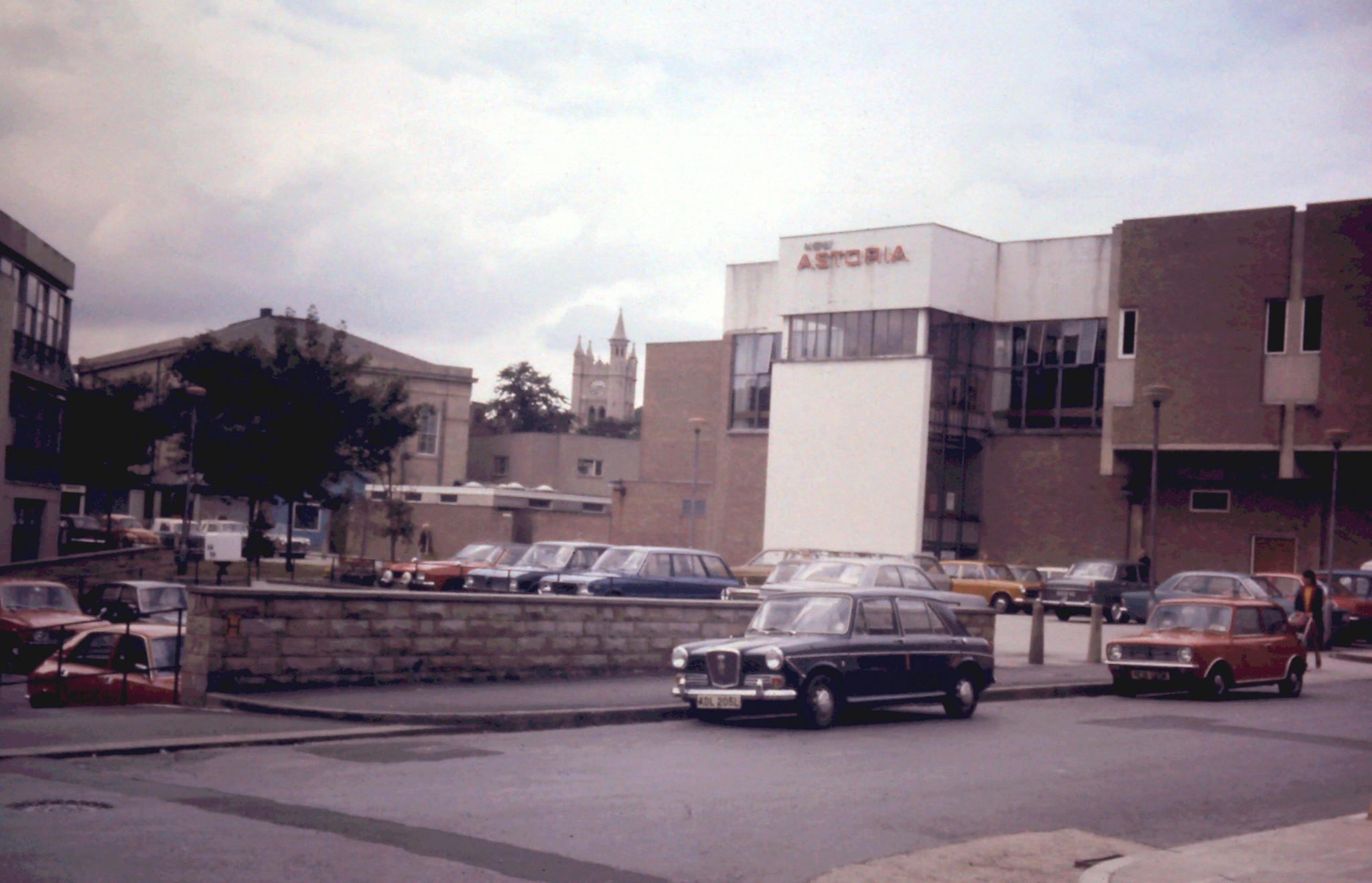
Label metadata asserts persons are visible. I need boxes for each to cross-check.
[416,523,436,560]
[1294,570,1325,668]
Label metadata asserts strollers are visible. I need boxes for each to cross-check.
[1286,611,1313,648]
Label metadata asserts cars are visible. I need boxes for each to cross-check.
[537,545,741,601]
[723,548,951,602]
[1103,598,1308,702]
[671,587,996,731]
[929,560,1068,615]
[1041,561,1149,624]
[462,540,611,594]
[80,580,187,665]
[0,576,112,678]
[27,623,186,709]
[381,541,531,592]
[60,512,161,554]
[151,518,310,558]
[1121,573,1268,630]
[1257,568,1372,649]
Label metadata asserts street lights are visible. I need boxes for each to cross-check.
[1145,383,1173,600]
[689,418,708,549]
[180,385,206,573]
[1324,428,1349,644]
